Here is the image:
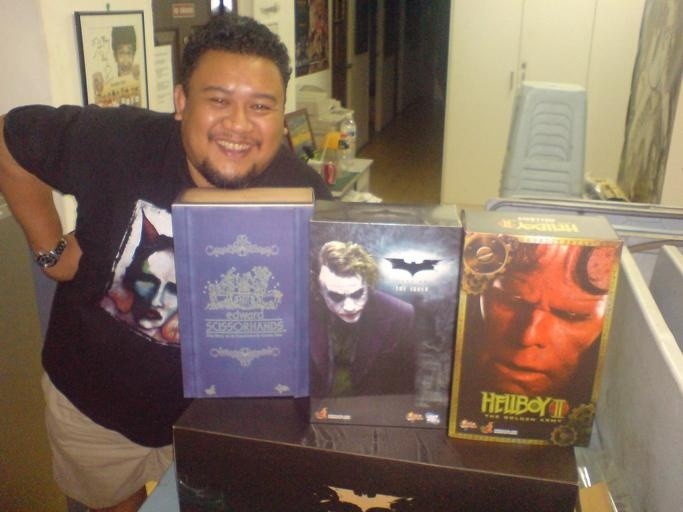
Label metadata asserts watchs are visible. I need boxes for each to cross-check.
[32,238,67,268]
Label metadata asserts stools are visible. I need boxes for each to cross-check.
[499,79,590,199]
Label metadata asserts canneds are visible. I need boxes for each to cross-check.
[326,162,337,186]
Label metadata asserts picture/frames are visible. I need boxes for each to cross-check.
[73,9,150,110]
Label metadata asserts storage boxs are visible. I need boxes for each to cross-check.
[171,396,580,511]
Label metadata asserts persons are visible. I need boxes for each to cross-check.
[318,241,417,394]
[112,26,137,78]
[481,239,609,396]
[113,231,180,344]
[0,16,330,511]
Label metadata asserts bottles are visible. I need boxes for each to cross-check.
[339,112,356,159]
[318,132,339,191]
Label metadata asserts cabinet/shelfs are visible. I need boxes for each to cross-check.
[331,158,374,201]
